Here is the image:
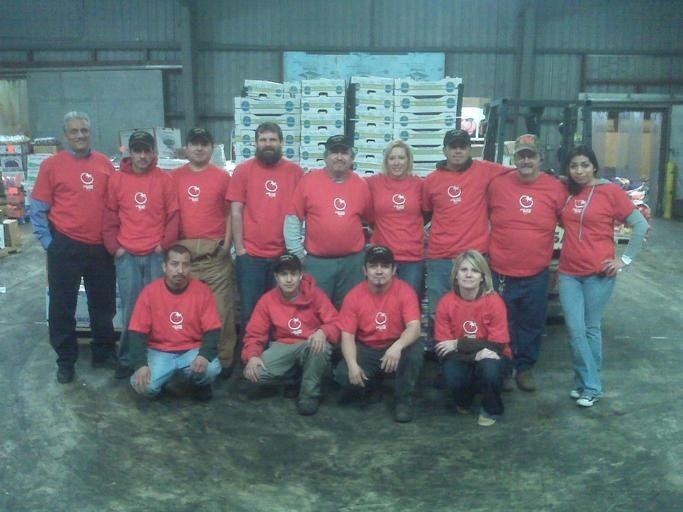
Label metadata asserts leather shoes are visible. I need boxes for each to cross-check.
[56,364,73,381]
[94,351,120,368]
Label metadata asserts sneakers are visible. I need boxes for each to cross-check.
[185,380,212,401]
[366,386,382,403]
[457,371,535,427]
[395,396,413,421]
[568,387,600,406]
[115,365,132,379]
[250,380,341,415]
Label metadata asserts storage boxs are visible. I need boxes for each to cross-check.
[43,284,122,330]
[229,71,466,179]
[117,128,182,161]
[0,131,65,258]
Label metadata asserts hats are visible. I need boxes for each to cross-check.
[326,135,353,150]
[129,131,154,148]
[444,129,471,146]
[188,127,212,143]
[514,134,537,153]
[366,245,392,262]
[273,254,300,271]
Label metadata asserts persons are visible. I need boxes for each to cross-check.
[225,121,303,337]
[127,245,222,402]
[169,127,237,380]
[486,134,573,392]
[420,129,515,358]
[29,111,115,385]
[558,144,648,408]
[242,253,341,415]
[102,131,180,380]
[433,248,513,417]
[283,134,371,310]
[336,246,425,422]
[364,138,425,307]
